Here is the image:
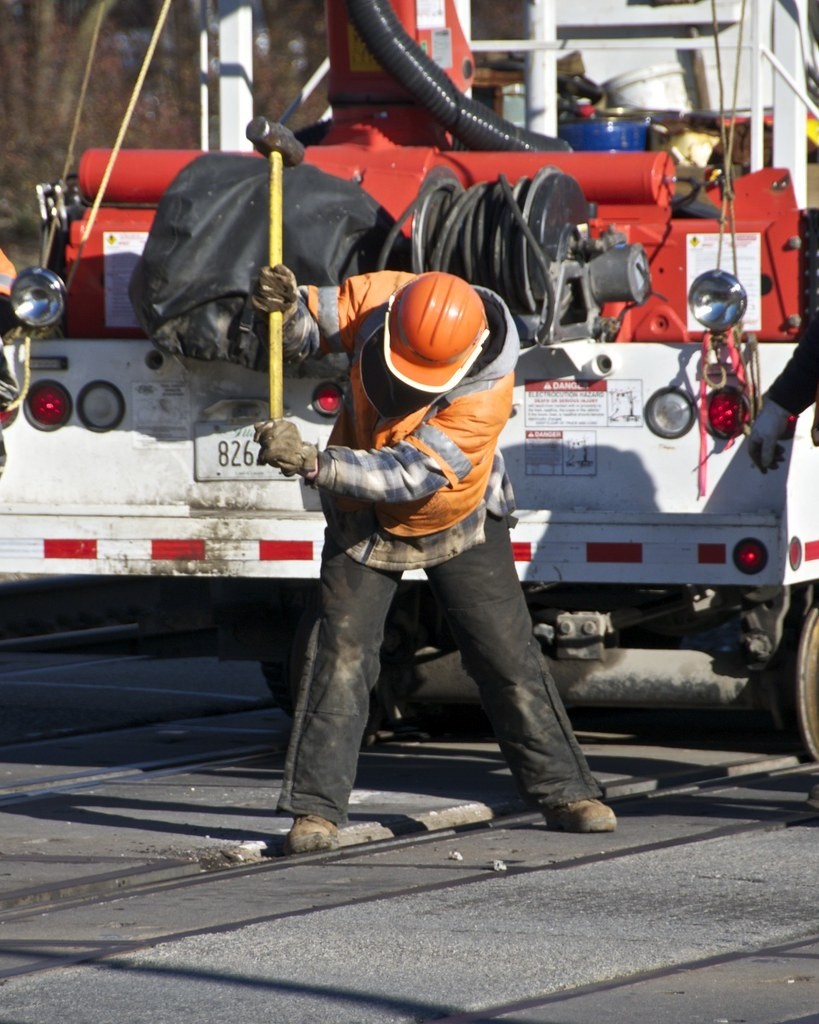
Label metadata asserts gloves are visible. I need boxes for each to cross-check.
[749,400,790,473]
[254,419,318,478]
[251,265,299,326]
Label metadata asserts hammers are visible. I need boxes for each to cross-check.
[245,115,302,424]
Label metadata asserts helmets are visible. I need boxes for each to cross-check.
[384,270,488,394]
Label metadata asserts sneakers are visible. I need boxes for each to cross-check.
[545,797,617,832]
[289,814,338,852]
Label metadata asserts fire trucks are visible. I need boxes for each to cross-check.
[0,0,819,768]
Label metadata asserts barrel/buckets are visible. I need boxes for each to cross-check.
[564,120,648,151]
[604,64,692,112]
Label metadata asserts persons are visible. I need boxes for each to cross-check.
[225,265,617,856]
